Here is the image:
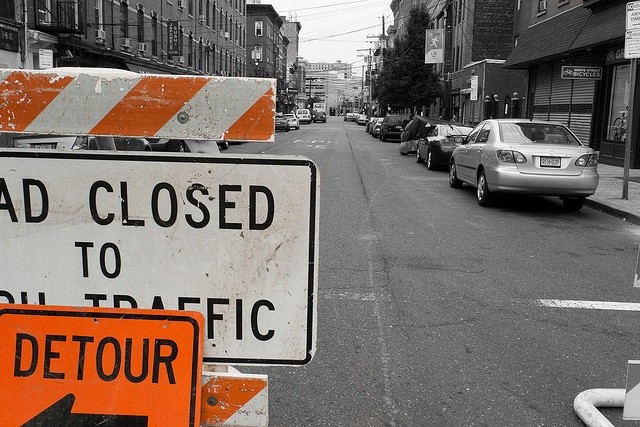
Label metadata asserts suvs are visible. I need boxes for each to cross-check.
[379,115,411,141]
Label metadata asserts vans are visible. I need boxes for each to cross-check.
[296,108,311,123]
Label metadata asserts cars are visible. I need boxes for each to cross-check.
[284,113,299,130]
[366,116,384,140]
[275,114,289,132]
[344,112,369,125]
[416,123,475,171]
[313,111,326,122]
[449,117,599,212]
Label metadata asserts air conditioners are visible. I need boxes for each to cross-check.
[138,43,148,52]
[200,15,206,21]
[178,56,184,64]
[96,30,106,39]
[121,38,133,48]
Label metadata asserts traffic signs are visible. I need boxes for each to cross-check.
[624,29,640,59]
[0,305,204,426]
[625,0,640,29]
